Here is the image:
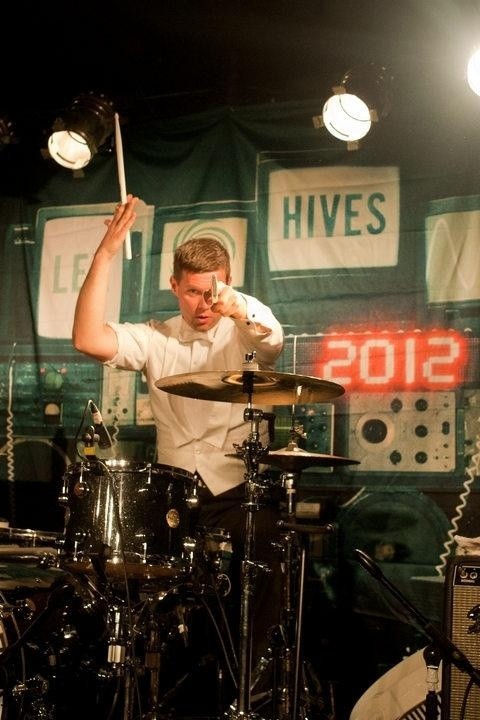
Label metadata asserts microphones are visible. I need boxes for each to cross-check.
[91,404,116,459]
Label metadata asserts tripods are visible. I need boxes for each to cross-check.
[59,563,206,720]
[228,458,335,720]
[214,430,275,720]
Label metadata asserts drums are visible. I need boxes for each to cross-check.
[1,526,58,591]
[60,460,196,578]
[192,527,231,597]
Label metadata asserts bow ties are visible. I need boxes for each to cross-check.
[178,328,216,345]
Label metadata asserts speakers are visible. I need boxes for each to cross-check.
[442,554,480,720]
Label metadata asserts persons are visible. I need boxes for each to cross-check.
[72,193,351,719]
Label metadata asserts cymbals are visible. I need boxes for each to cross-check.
[156,372,345,404]
[226,444,361,468]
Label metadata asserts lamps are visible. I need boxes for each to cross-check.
[313,67,378,150]
[41,90,117,169]
